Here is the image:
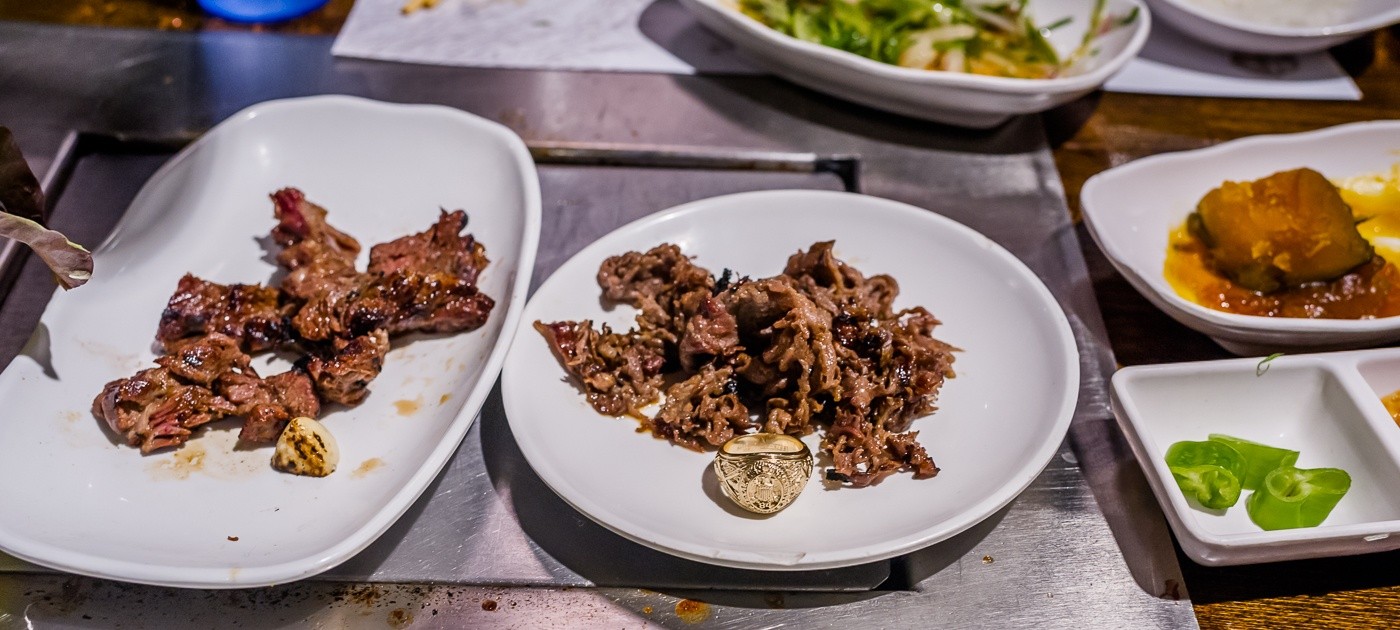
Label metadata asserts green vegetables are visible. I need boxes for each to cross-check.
[737,0,1139,67]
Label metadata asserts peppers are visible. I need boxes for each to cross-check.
[1164,433,1351,531]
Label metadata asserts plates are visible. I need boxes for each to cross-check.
[0,94,540,591]
[1147,0,1400,54]
[503,190,1081,572]
[1081,120,1400,342]
[1109,347,1399,566]
[683,0,1152,129]
